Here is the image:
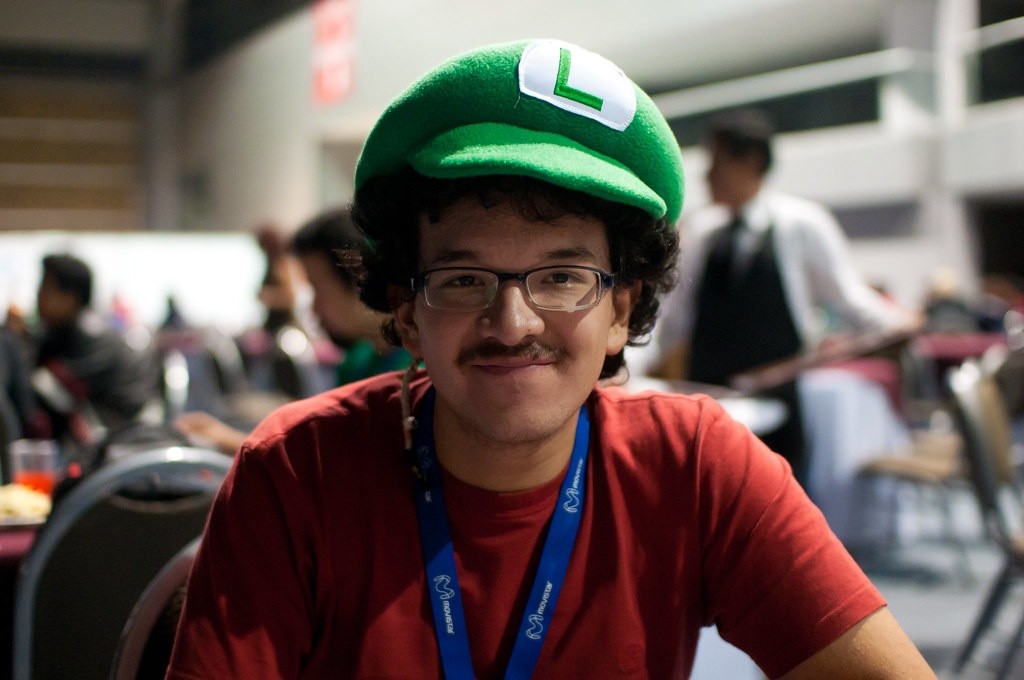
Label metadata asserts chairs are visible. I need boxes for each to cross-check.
[13,345,1024,680]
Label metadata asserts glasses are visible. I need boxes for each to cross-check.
[411,264,619,313]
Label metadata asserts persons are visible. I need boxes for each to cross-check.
[159,39,936,680]
[1,211,422,471]
[661,108,913,491]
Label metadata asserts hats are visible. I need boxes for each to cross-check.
[355,41,685,262]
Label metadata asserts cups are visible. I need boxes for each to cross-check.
[7,441,56,502]
